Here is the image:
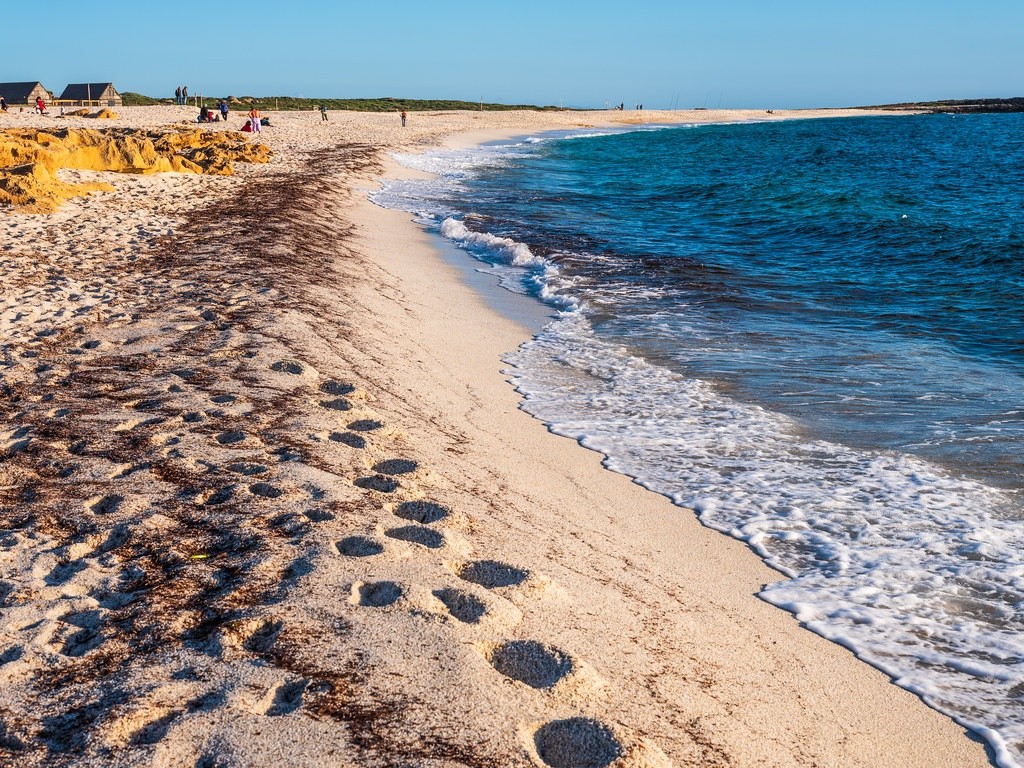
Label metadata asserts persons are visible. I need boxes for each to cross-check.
[240,107,269,132]
[0,95,7,110]
[197,103,214,123]
[175,87,183,105]
[400,110,406,127]
[33,96,46,113]
[220,101,228,120]
[182,86,187,105]
[321,104,328,121]
